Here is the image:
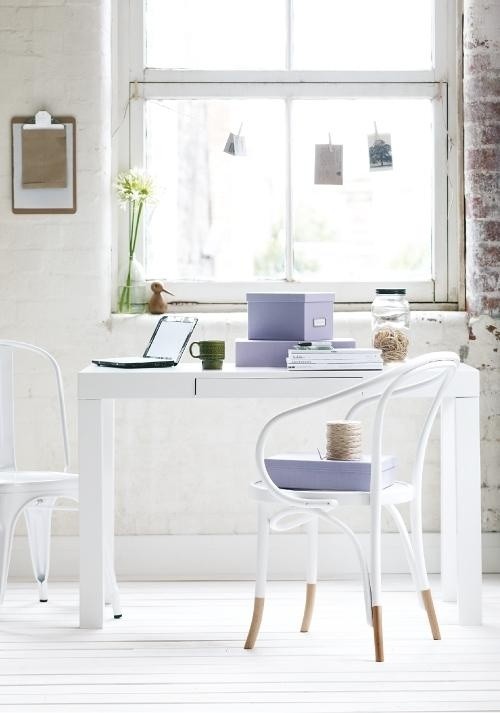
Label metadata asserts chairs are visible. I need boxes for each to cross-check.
[1,340,123,619]
[242,350,460,663]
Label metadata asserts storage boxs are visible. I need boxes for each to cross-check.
[235,292,356,366]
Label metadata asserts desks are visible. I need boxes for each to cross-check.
[77,358,481,629]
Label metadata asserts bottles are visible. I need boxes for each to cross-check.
[371,287,410,362]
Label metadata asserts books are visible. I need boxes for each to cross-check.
[286,340,385,372]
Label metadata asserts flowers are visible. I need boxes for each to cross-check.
[113,167,162,313]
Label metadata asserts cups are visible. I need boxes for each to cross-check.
[188,339,226,370]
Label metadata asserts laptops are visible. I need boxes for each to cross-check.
[90,316,198,368]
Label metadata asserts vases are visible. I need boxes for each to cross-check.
[115,253,149,313]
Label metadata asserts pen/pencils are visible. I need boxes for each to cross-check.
[298,341,331,346]
